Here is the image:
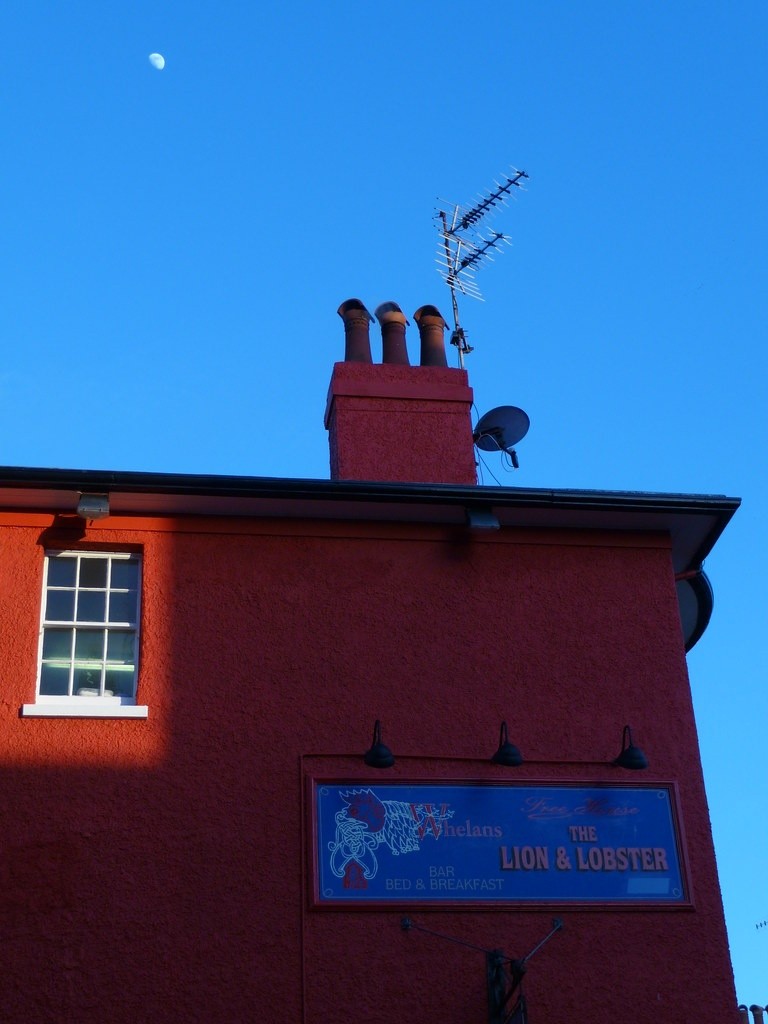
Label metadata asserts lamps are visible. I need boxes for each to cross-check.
[365,719,396,768]
[612,725,650,770]
[466,513,502,540]
[490,720,525,767]
[76,494,110,521]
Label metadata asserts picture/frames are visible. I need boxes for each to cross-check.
[308,774,697,911]
